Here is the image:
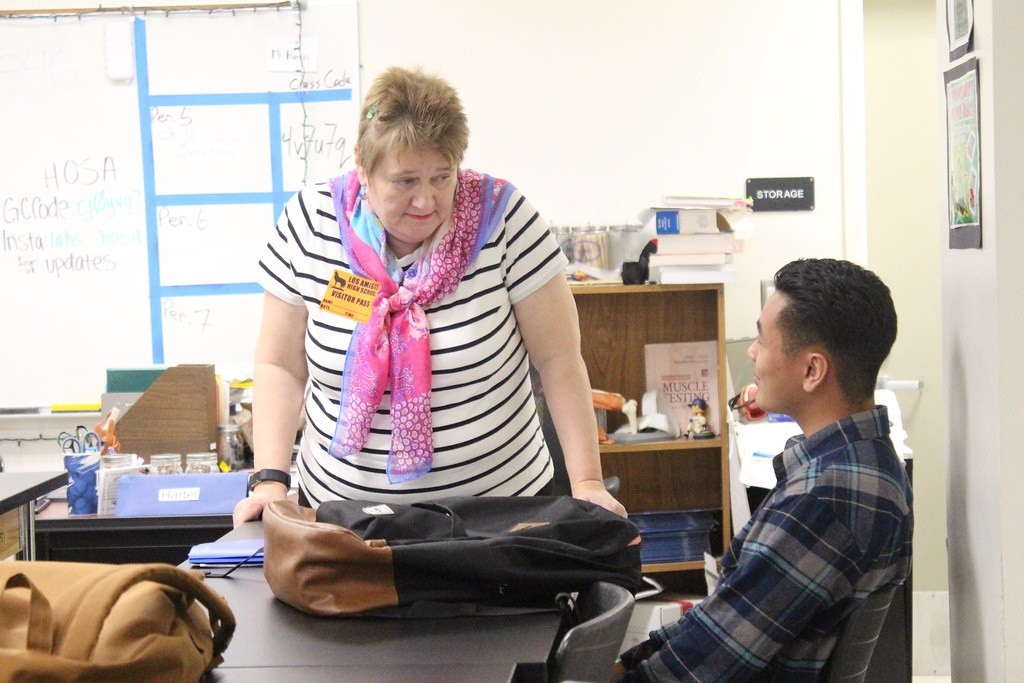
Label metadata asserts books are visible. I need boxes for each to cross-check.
[638,198,750,284]
[628,507,720,564]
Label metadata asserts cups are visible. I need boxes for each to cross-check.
[62,453,99,515]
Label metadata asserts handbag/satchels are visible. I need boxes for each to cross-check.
[0,559,236,683]
[58,425,102,515]
[91,407,122,456]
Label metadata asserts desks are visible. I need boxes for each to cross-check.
[170,537,571,682]
[0,465,69,560]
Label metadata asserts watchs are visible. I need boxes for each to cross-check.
[247,468,291,490]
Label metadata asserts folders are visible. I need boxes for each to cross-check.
[116,472,249,517]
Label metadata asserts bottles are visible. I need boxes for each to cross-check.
[149,454,183,475]
[216,425,244,468]
[184,453,219,474]
[549,224,640,276]
[102,453,133,469]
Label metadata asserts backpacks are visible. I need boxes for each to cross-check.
[262,494,645,616]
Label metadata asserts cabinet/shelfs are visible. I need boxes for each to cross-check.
[569,280,729,575]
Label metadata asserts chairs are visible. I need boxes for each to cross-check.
[553,579,638,683]
[824,584,898,681]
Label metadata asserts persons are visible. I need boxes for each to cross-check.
[604,256,915,683]
[232,68,628,550]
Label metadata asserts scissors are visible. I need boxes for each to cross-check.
[62,431,101,453]
[57,424,88,453]
